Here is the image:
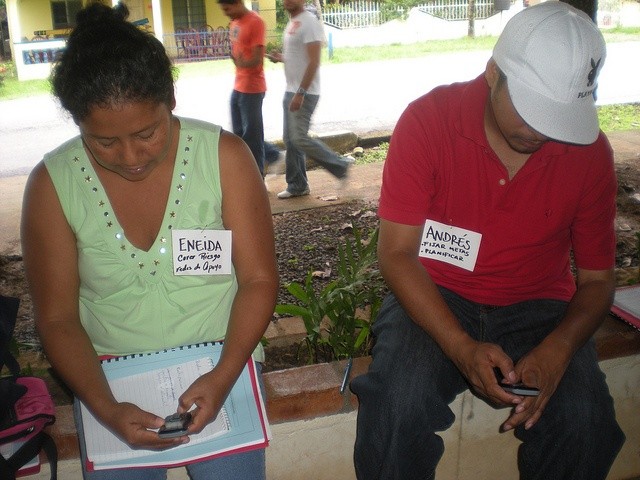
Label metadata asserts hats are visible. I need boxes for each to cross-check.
[493,0,607,145]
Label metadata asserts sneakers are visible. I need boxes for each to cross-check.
[277,186,310,199]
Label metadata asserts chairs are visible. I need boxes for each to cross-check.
[199,24,214,57]
[176,27,186,58]
[31,36,46,41]
[186,27,199,58]
[214,26,226,57]
[226,22,232,56]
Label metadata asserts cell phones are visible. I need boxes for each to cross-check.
[157,413,191,439]
[500,384,540,398]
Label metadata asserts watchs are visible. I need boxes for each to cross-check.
[298,88,305,93]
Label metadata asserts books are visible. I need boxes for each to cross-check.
[77,338,270,475]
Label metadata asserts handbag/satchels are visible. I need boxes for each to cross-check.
[0,376,56,445]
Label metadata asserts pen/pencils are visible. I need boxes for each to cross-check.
[339,358,352,393]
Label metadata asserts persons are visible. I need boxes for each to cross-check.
[19,4,277,480]
[214,0,288,191]
[264,0,355,199]
[347,0,625,478]
